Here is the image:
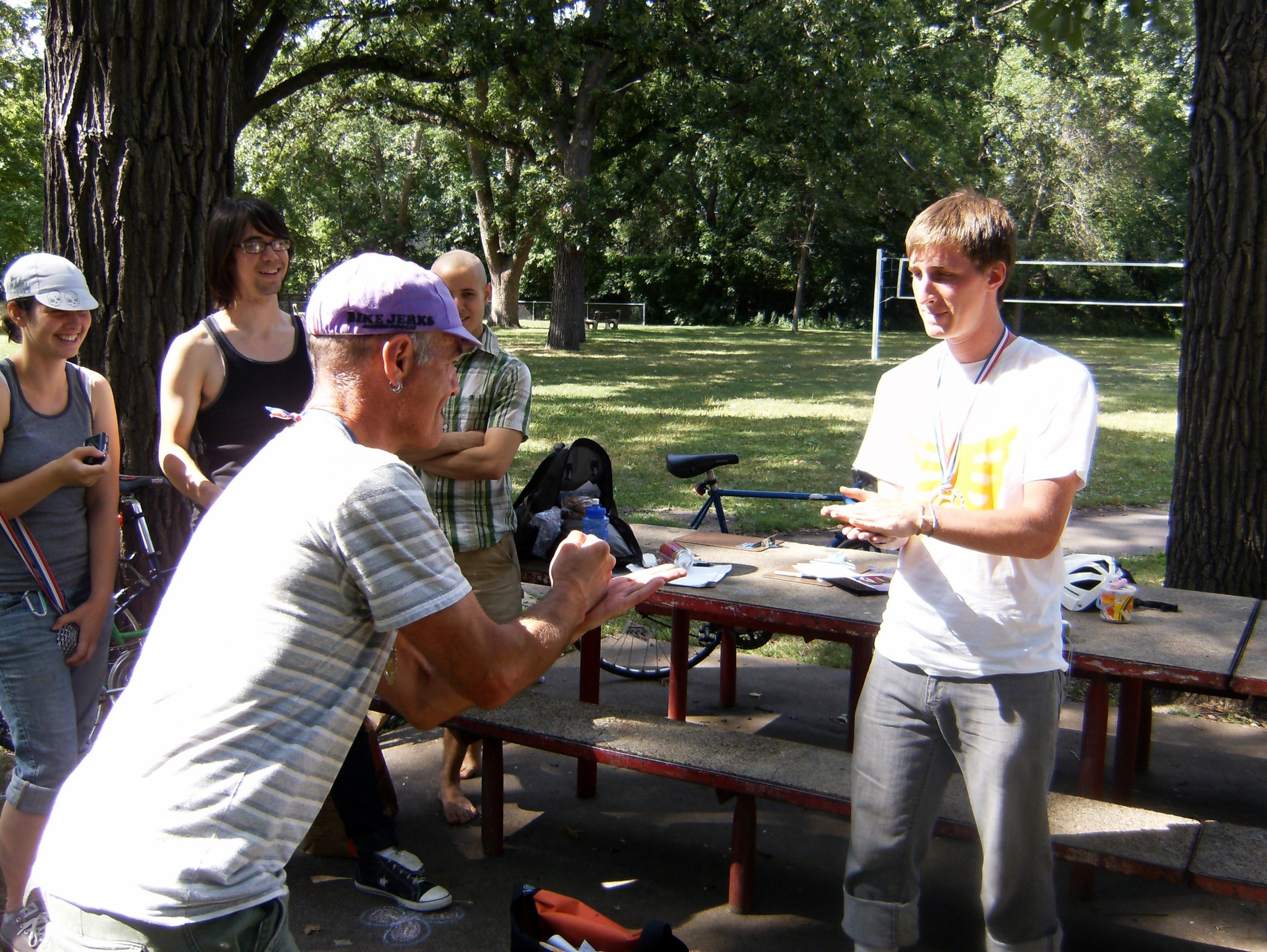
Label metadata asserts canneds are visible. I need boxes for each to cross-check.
[660,541,694,570]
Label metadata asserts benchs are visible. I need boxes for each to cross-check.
[585,309,622,330]
[366,684,1267,914]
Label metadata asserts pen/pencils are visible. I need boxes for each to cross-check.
[774,570,816,579]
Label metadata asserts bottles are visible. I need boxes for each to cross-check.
[582,497,610,542]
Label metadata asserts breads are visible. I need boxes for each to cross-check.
[563,495,593,520]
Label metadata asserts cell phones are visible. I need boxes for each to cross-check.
[83,432,109,463]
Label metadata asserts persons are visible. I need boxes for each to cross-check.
[396,251,531,823]
[156,194,452,912]
[0,253,119,952]
[819,194,1100,952]
[22,251,686,952]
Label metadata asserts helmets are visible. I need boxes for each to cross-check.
[1062,552,1128,611]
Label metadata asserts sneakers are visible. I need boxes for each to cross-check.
[0,902,51,952]
[353,849,450,911]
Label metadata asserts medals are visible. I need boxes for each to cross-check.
[929,486,964,508]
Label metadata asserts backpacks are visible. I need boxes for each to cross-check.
[513,439,643,573]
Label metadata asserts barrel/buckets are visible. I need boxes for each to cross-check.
[1096,582,1137,624]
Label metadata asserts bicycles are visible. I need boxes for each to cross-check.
[0,473,180,754]
[569,454,884,681]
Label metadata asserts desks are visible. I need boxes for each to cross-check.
[517,487,1267,803]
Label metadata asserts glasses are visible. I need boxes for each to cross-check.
[234,239,291,254]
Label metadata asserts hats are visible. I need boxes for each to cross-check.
[304,251,484,349]
[4,252,98,309]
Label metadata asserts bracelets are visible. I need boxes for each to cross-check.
[915,505,925,535]
[927,506,938,538]
